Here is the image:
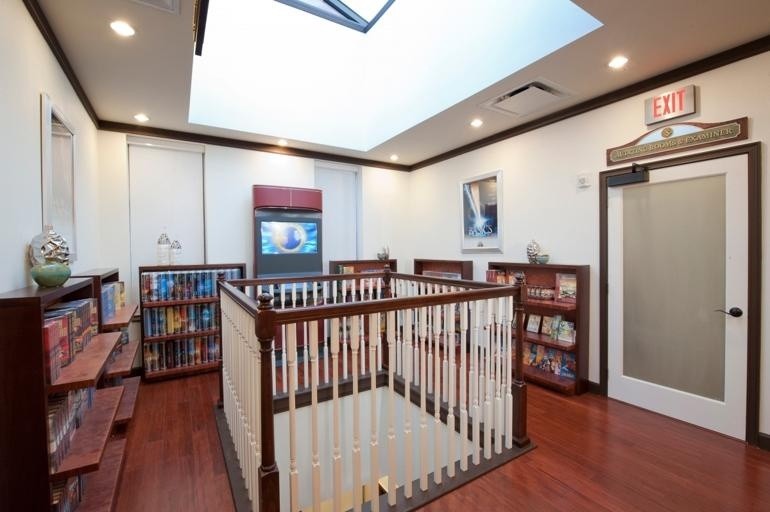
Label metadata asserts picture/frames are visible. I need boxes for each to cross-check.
[459,168,503,254]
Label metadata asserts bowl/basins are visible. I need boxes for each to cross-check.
[535,255,550,265]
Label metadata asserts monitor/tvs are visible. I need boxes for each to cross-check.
[253,215,322,275]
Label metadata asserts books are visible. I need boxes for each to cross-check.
[485,269,578,380]
[43,281,129,512]
[333,264,392,344]
[141,268,244,373]
[418,269,463,346]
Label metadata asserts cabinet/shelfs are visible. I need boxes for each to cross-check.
[140,267,244,376]
[4,267,138,509]
[329,259,590,397]
